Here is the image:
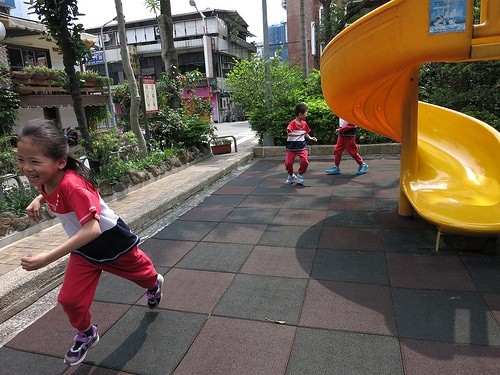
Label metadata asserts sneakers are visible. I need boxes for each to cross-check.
[325,166,340,174]
[145,273,164,309]
[285,174,296,184]
[296,174,304,184]
[64,323,100,366]
[356,163,368,175]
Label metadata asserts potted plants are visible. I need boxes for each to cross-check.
[22,65,33,79]
[50,68,59,81]
[85,70,99,84]
[33,65,52,81]
[211,139,232,155]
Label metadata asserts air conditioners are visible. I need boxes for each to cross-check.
[103,34,109,42]
[155,27,160,36]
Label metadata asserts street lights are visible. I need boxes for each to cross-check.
[100,15,126,127]
[188,0,215,125]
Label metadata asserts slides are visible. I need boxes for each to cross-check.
[320,0,500,253]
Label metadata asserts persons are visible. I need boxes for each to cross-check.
[17,118,164,365]
[436,15,455,29]
[285,103,317,185]
[325,115,369,176]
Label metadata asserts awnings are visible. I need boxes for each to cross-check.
[0,13,98,53]
[11,94,112,108]
